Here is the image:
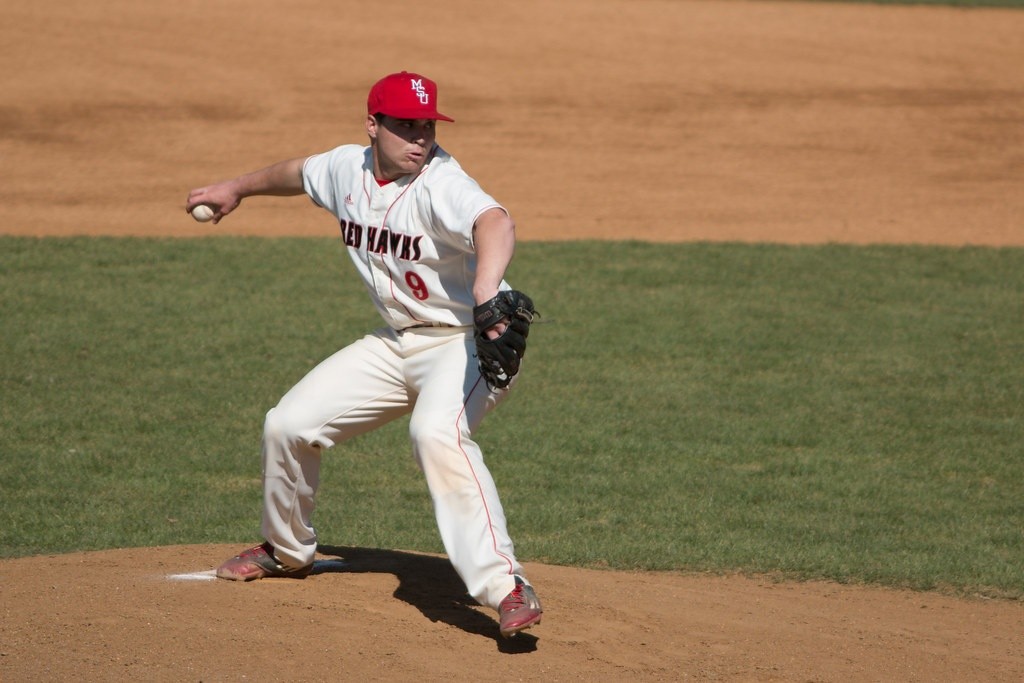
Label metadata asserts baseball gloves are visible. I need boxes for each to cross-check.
[470,289,543,396]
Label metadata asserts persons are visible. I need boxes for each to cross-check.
[184,70,546,640]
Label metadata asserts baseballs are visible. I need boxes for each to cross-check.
[191,204,216,223]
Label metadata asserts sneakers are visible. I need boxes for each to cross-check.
[215,540,316,582]
[498,581,542,640]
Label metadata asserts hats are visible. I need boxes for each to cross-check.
[366,70,455,124]
